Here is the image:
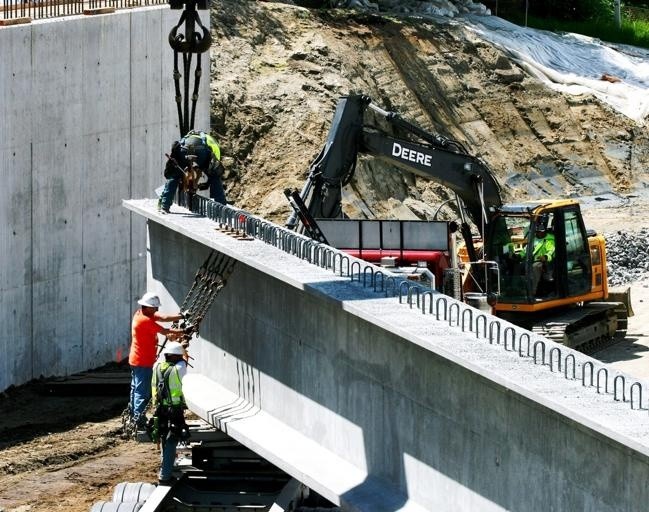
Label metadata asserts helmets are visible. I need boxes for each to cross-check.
[164,342,186,355]
[137,292,161,307]
[535,225,546,232]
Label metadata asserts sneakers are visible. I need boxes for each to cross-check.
[171,466,180,472]
[158,476,178,484]
[161,208,170,213]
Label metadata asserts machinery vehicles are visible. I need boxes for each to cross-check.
[286,90,634,356]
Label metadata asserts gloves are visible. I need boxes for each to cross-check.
[183,325,194,333]
[199,182,208,190]
[179,309,191,319]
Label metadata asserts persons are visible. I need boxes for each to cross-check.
[149,334,187,485]
[520,224,558,299]
[158,128,231,216]
[127,290,188,425]
